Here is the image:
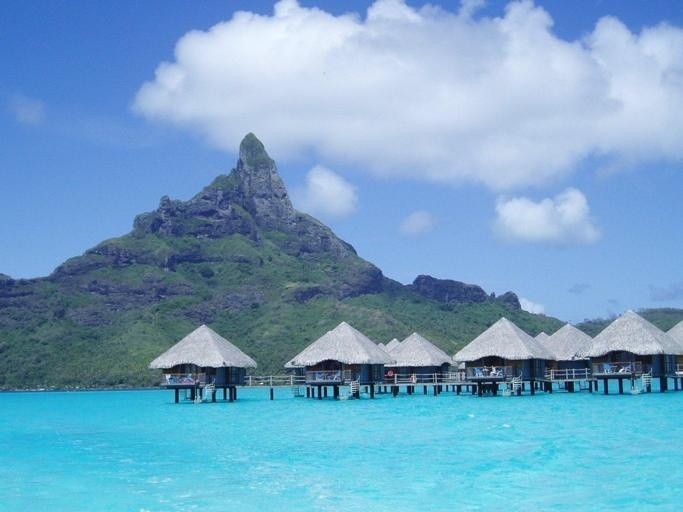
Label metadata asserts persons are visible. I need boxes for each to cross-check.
[601,362,632,373]
[165,372,198,385]
[473,366,502,376]
